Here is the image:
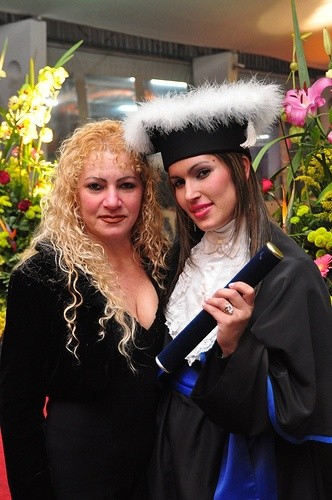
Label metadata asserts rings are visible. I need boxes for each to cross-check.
[224,303,235,315]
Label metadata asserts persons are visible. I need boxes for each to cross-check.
[117,70,331,500]
[0,118,171,500]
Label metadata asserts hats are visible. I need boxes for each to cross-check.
[119,72,286,172]
